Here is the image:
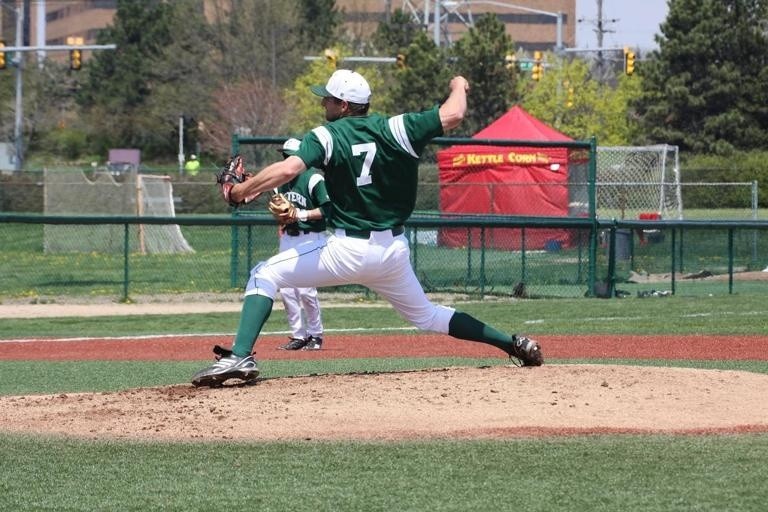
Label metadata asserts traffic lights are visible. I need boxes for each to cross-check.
[396,55,405,69]
[624,50,635,78]
[325,50,338,67]
[564,79,575,109]
[532,52,543,83]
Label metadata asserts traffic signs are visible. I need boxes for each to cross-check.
[520,63,532,71]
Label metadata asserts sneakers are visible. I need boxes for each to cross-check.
[190,345,260,387]
[301,336,323,350]
[512,335,545,367]
[284,337,306,350]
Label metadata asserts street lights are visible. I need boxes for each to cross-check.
[439,0,565,105]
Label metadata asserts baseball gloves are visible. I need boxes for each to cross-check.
[215,154,263,209]
[267,193,299,224]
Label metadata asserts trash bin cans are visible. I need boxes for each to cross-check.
[606,227,632,260]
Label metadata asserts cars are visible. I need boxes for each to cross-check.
[87,159,153,185]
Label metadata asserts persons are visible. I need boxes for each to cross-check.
[190,68,542,388]
[268,139,329,350]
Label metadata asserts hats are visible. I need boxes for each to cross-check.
[276,138,301,151]
[307,69,374,106]
